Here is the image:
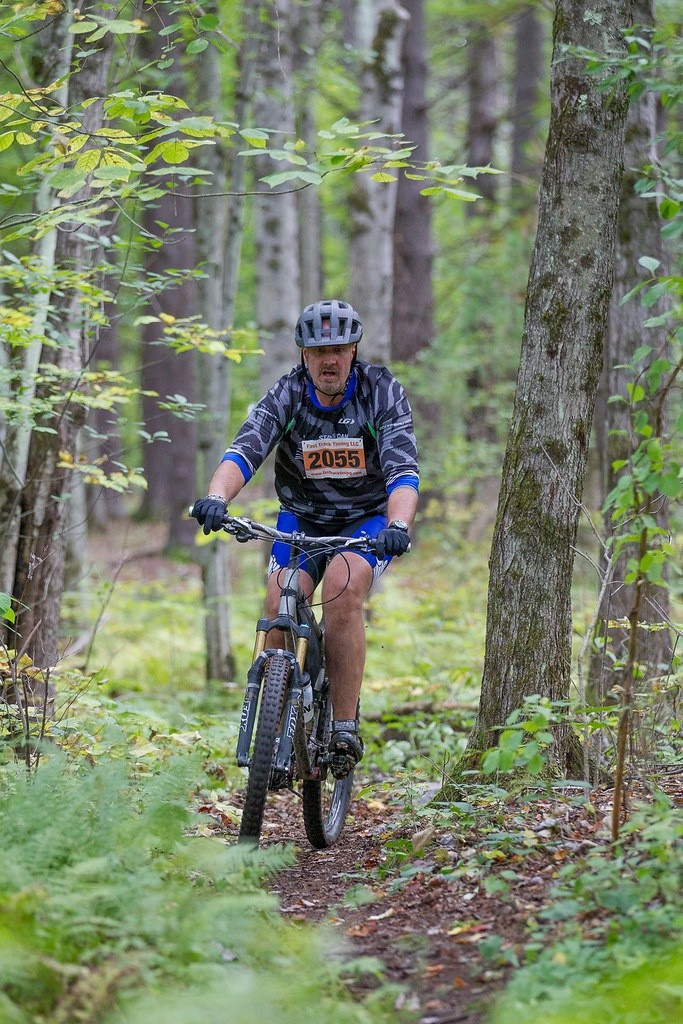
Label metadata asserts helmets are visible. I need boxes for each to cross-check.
[294,300,363,348]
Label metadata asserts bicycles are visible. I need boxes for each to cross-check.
[184,501,413,855]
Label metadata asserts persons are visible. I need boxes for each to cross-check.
[192,299,420,780]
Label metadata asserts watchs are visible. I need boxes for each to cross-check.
[388,520,408,534]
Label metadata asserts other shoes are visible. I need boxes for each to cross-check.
[328,719,363,779]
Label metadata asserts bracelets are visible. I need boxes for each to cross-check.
[206,494,226,506]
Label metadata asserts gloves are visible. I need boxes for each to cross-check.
[193,498,226,535]
[370,527,410,561]
